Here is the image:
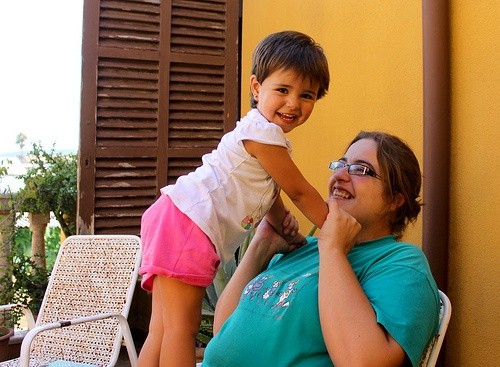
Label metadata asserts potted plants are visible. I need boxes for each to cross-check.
[0,187,54,362]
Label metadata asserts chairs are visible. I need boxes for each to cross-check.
[0,233,142,367]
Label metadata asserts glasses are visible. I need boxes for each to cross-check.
[328,160,385,182]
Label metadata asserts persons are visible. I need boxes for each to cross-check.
[199,128,441,367]
[133,30,330,367]
